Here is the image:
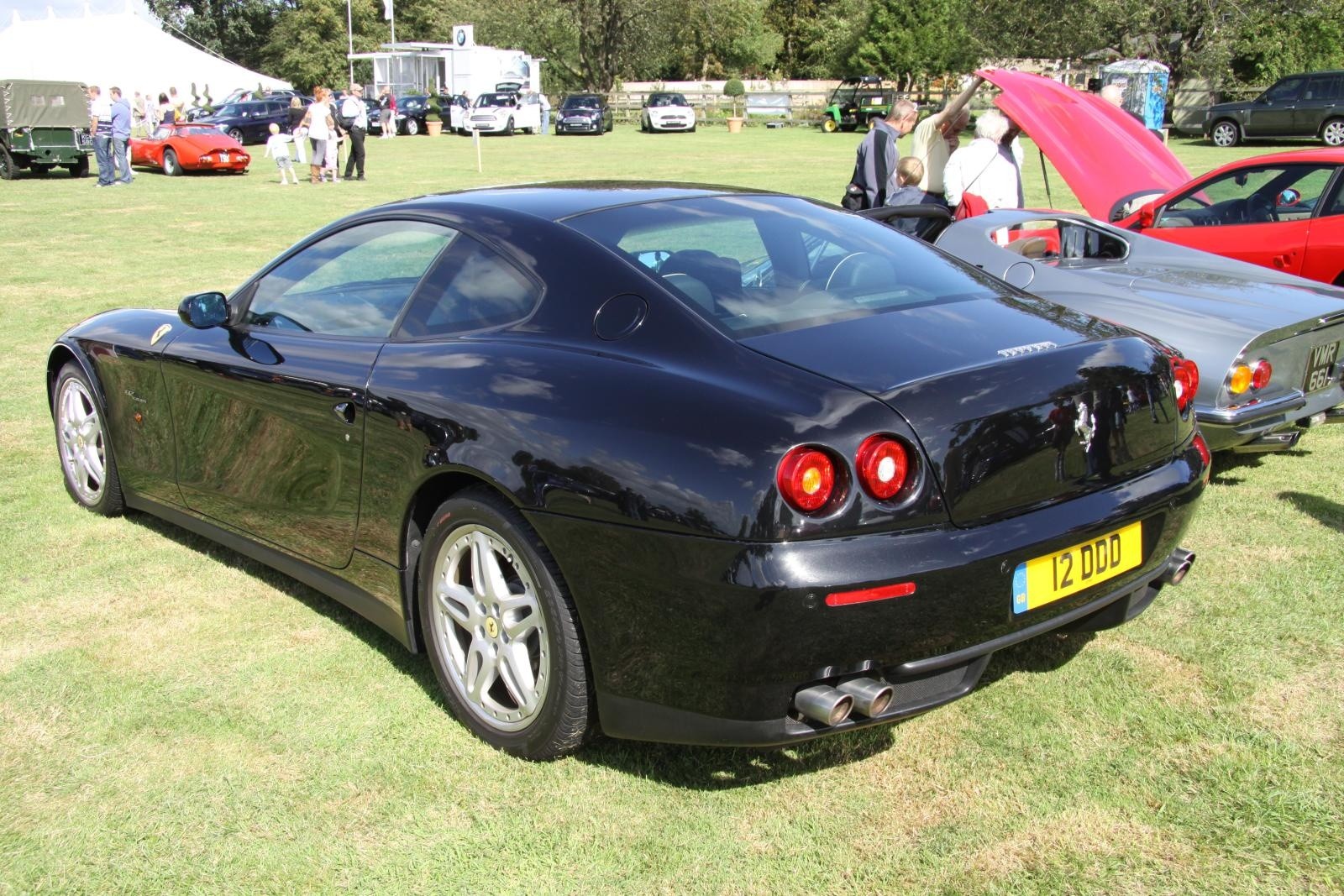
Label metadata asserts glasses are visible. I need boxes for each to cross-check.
[948,119,961,133]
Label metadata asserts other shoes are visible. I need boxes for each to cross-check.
[357,175,365,181]
[344,176,352,180]
[280,181,287,185]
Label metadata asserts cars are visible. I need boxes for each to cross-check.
[450,82,541,137]
[185,87,384,146]
[554,92,613,136]
[640,92,697,134]
[366,94,457,137]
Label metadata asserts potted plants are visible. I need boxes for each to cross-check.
[423,96,444,137]
[723,78,744,133]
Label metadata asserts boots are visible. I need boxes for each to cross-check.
[311,165,319,184]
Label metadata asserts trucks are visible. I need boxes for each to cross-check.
[0,76,96,181]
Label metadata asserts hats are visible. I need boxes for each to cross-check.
[350,83,363,91]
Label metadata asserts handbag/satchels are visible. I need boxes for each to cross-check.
[841,183,866,211]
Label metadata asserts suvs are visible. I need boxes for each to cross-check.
[1201,70,1344,149]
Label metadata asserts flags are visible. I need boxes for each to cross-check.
[384,0,393,23]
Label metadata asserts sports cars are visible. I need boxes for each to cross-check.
[112,122,251,177]
[44,175,1215,766]
[947,66,1344,290]
[738,198,1344,458]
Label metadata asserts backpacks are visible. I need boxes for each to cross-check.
[335,99,360,128]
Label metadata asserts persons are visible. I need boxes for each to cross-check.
[145,95,159,129]
[320,114,346,183]
[264,123,302,185]
[291,97,302,107]
[881,157,951,245]
[519,89,533,104]
[295,86,334,184]
[88,86,115,188]
[109,87,133,185]
[238,94,248,101]
[942,111,1024,231]
[455,90,470,135]
[342,83,367,181]
[170,87,185,113]
[377,85,398,139]
[443,87,451,96]
[154,92,174,124]
[913,77,984,213]
[532,92,552,134]
[132,108,142,137]
[998,109,1024,171]
[850,99,918,233]
[135,92,151,136]
[339,87,349,99]
